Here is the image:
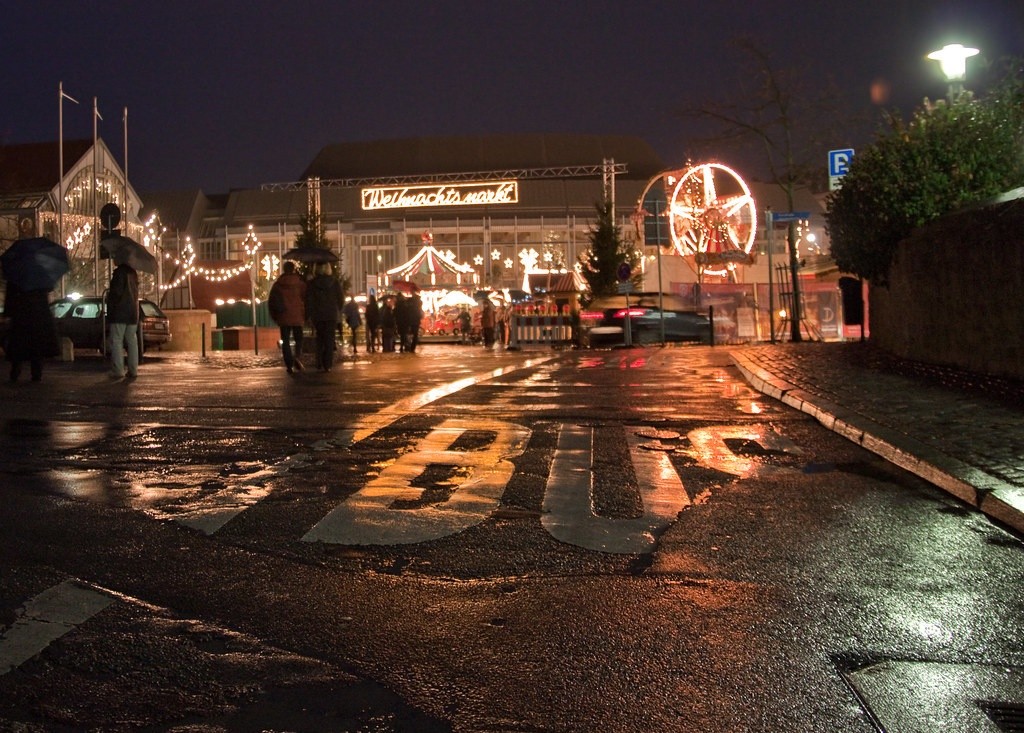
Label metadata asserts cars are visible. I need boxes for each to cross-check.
[43,296,173,359]
[417,313,460,335]
[580,293,727,345]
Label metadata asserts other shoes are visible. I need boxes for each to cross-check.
[287,368,292,372]
[317,364,321,369]
[125,373,137,378]
[108,373,123,380]
[31,377,41,382]
[325,367,331,372]
[294,359,303,370]
[8,379,17,384]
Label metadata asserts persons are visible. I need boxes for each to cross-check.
[482,301,519,347]
[0,280,50,384]
[105,258,139,377]
[307,263,345,373]
[364,288,423,353]
[453,308,475,346]
[343,293,362,353]
[268,262,311,373]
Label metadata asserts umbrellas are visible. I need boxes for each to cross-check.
[100,236,159,275]
[281,248,343,263]
[376,295,396,302]
[0,237,72,293]
[389,280,420,293]
